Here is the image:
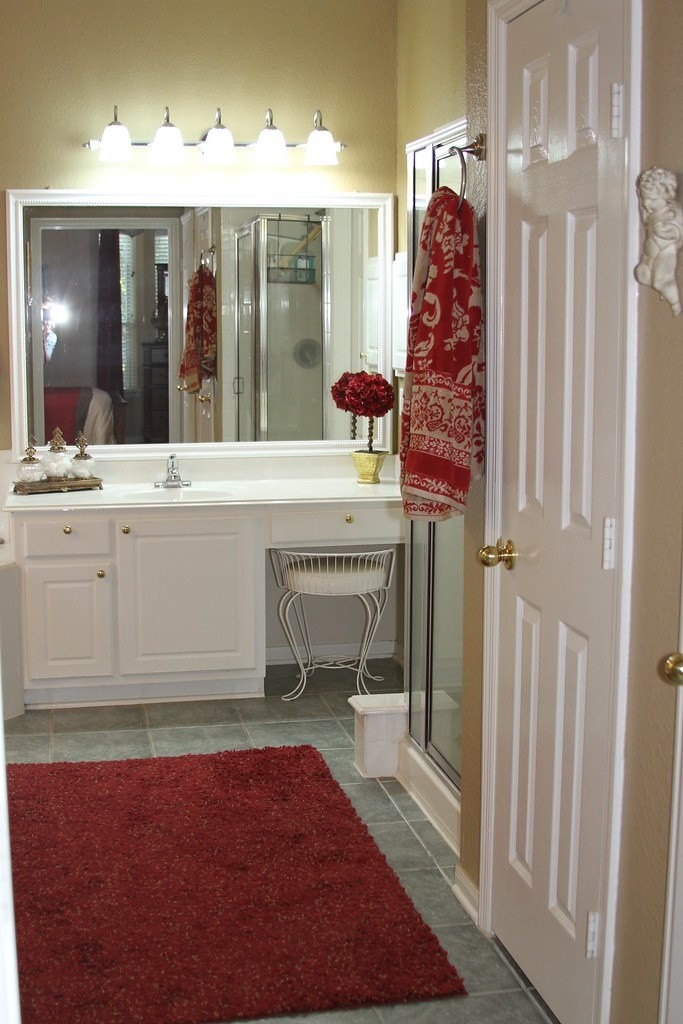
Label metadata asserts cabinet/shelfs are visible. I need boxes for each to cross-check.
[11,502,409,711]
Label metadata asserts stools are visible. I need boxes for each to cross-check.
[269,548,397,701]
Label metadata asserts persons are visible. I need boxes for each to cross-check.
[635,166,683,315]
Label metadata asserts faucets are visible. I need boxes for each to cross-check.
[155,454,192,488]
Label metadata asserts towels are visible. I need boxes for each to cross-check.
[176,263,217,395]
[398,184,487,524]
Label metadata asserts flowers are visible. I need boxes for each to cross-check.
[345,371,395,452]
[329,371,371,440]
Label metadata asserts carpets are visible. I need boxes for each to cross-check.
[5,743,468,1024]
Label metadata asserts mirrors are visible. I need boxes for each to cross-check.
[5,189,396,460]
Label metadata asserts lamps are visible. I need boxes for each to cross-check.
[248,109,289,170]
[71,430,94,480]
[98,105,134,163]
[303,109,340,167]
[41,427,71,481]
[17,434,44,482]
[198,107,236,169]
[146,106,187,170]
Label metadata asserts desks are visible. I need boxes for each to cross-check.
[140,343,171,444]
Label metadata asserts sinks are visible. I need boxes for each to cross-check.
[125,486,234,503]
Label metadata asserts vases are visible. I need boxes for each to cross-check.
[351,450,388,484]
